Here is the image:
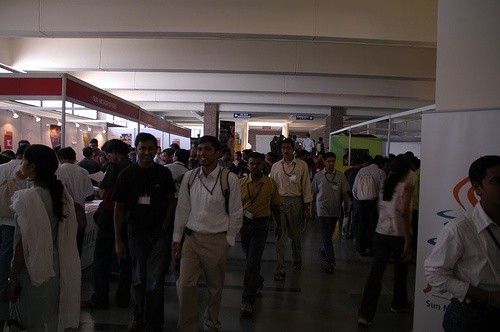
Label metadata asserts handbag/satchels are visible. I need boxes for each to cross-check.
[3,302,27,332]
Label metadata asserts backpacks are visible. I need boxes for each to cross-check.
[357,175,377,201]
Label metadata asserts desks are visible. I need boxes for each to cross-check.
[82,200,102,269]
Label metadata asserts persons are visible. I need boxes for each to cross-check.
[0,132,420,282]
[356,154,414,325]
[172,135,242,331]
[0,145,29,301]
[240,152,282,316]
[424,155,500,332]
[6,144,81,332]
[82,138,139,309]
[109,132,176,332]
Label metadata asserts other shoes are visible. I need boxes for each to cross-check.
[390,302,413,313]
[126,312,142,330]
[327,265,334,274]
[356,318,373,326]
[255,275,264,295]
[291,261,302,271]
[239,303,252,314]
[274,271,284,280]
[81,301,94,308]
[319,248,328,263]
